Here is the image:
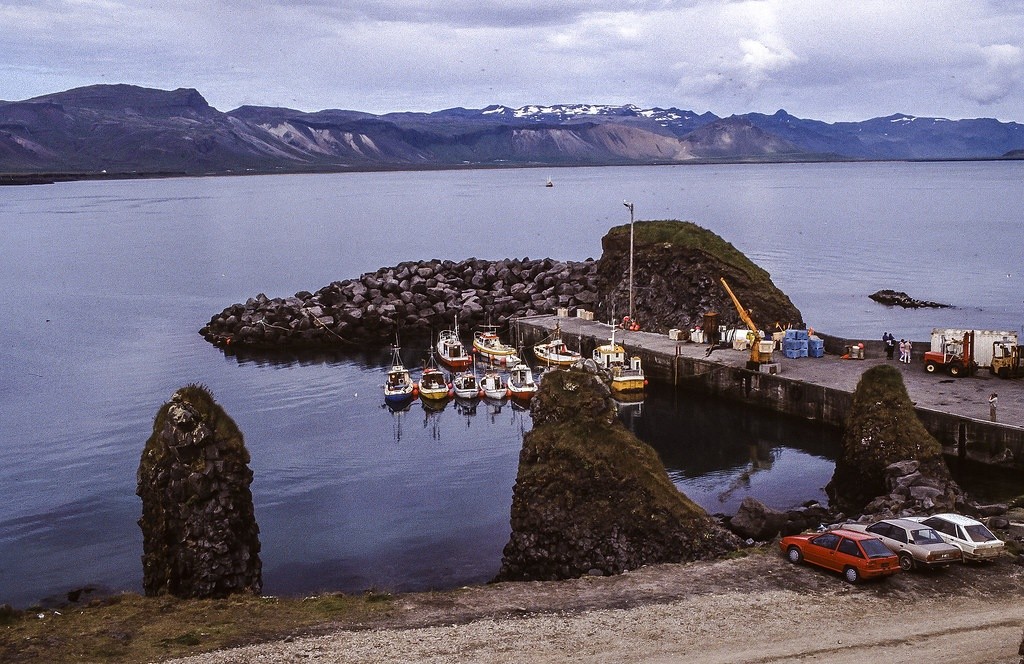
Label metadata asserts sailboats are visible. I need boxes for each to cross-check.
[546,174,552,186]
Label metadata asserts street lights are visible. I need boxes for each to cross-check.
[623,201,634,319]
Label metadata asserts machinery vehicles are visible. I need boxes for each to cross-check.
[924,330,992,378]
[990,340,1024,378]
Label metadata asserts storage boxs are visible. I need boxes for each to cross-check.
[758,330,824,358]
[669,329,682,340]
[577,309,593,320]
[691,332,704,343]
[558,308,568,317]
[852,346,860,358]
[733,341,746,349]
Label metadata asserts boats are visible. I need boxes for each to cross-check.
[437,357,469,376]
[438,328,471,367]
[478,368,511,400]
[472,316,521,363]
[507,363,539,403]
[451,371,479,399]
[385,344,414,404]
[418,330,450,400]
[592,319,649,392]
[610,390,645,417]
[534,327,582,366]
[382,398,533,442]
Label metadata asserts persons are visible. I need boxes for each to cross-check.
[899,339,912,363]
[989,393,998,422]
[882,332,896,360]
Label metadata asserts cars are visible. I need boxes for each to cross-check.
[903,513,1005,562]
[779,528,902,583]
[840,518,963,574]
